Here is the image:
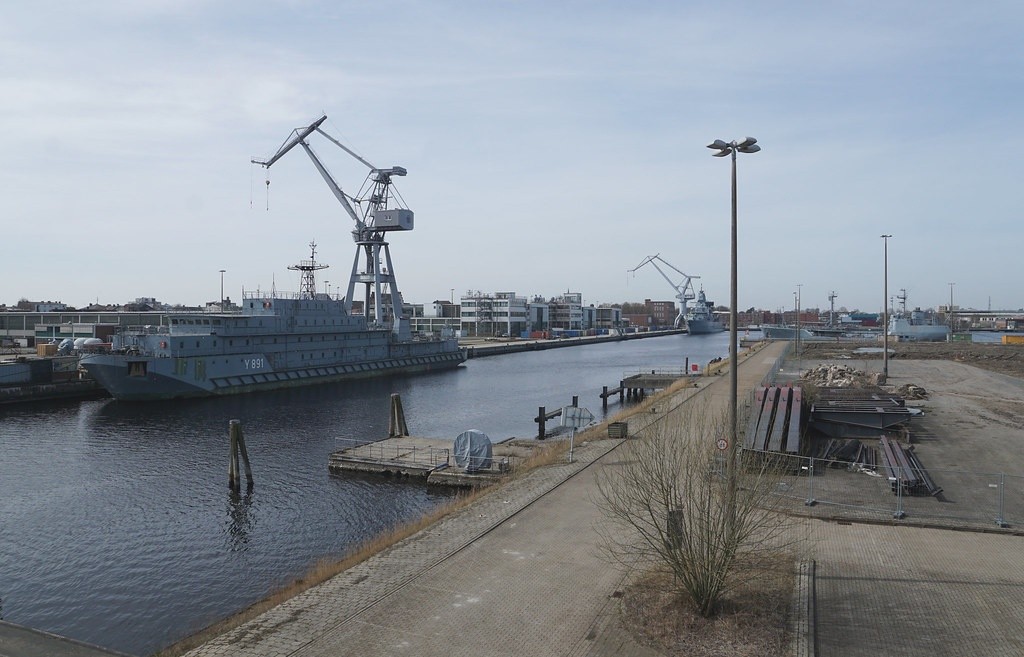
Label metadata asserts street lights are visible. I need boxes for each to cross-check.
[879,234,893,376]
[708,136,760,569]
[220,269,227,313]
[69,322,74,341]
[450,289,455,337]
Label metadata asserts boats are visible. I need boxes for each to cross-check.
[890,290,952,342]
[78,291,470,402]
[761,284,864,341]
[684,286,725,335]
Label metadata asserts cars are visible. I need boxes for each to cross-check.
[502,333,508,337]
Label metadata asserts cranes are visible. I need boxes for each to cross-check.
[251,114,414,329]
[627,253,701,328]
